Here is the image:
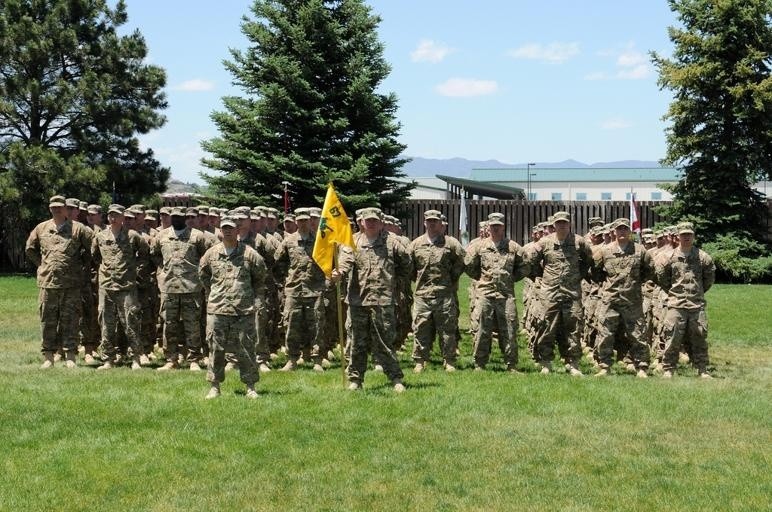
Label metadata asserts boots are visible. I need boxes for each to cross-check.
[225,361,238,371]
[506,364,523,372]
[392,377,406,393]
[534,361,552,374]
[564,357,582,376]
[259,344,336,372]
[204,382,220,400]
[582,346,713,378]
[413,358,426,373]
[38,343,209,370]
[474,362,485,370]
[348,379,362,389]
[443,358,455,371]
[245,382,262,399]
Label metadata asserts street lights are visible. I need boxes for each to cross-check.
[529,173,536,200]
[527,162,535,200]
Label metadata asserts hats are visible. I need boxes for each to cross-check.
[479,212,505,229]
[640,222,694,244]
[588,216,630,238]
[355,207,401,227]
[283,207,323,222]
[423,209,449,225]
[49,195,280,228]
[532,211,571,233]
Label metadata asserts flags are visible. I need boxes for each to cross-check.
[313,183,358,386]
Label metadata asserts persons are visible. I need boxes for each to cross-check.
[197,216,265,400]
[332,208,412,394]
[25,195,715,380]
